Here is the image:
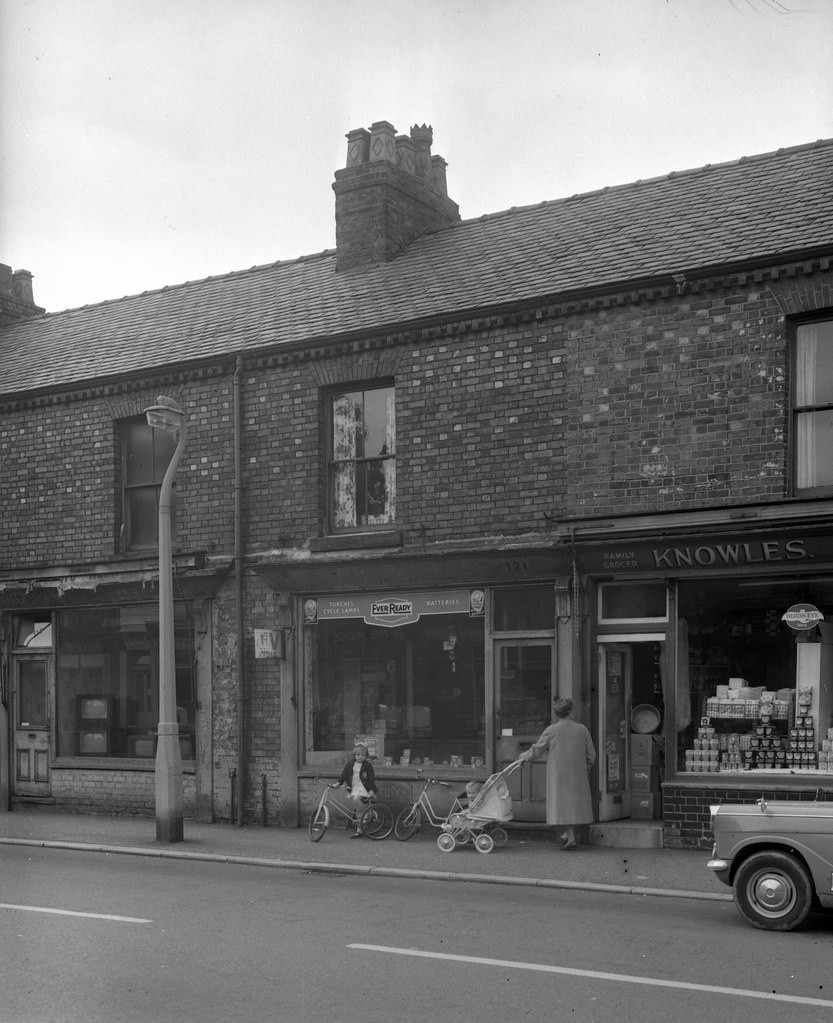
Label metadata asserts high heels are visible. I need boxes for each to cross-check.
[557,836,567,843]
[561,841,578,850]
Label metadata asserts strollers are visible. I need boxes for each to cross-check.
[437,759,523,856]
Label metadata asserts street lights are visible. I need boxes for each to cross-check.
[144,395,191,840]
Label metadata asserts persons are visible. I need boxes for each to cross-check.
[441,781,483,832]
[518,697,596,850]
[331,742,378,839]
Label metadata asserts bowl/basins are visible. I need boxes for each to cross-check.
[631,704,660,734]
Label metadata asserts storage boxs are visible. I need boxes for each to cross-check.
[628,733,664,819]
[686,679,833,773]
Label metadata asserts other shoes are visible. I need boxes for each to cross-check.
[352,832,364,837]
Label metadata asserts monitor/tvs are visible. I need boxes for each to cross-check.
[75,693,120,757]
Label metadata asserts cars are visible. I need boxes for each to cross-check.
[705,788,833,932]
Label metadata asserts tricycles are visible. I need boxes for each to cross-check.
[308,769,396,844]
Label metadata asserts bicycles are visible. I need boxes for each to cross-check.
[394,768,489,845]
